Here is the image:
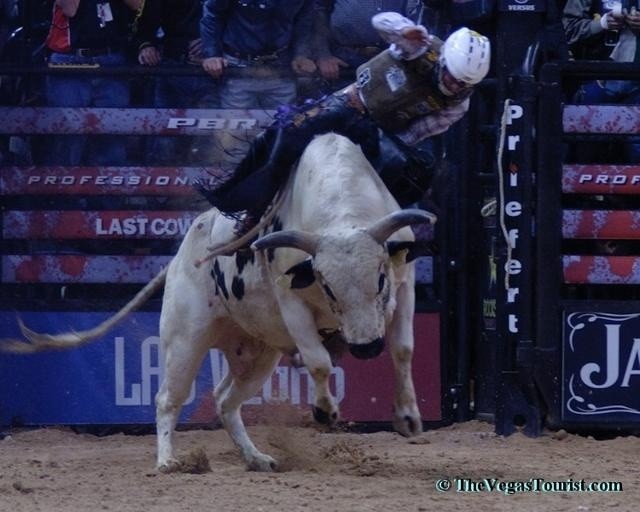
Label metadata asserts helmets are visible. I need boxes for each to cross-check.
[435,27,491,98]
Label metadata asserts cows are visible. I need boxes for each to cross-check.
[0,128,437,475]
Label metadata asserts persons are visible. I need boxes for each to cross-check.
[192,12,492,217]
[2,2,639,168]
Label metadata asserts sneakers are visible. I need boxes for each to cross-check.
[207,229,253,255]
